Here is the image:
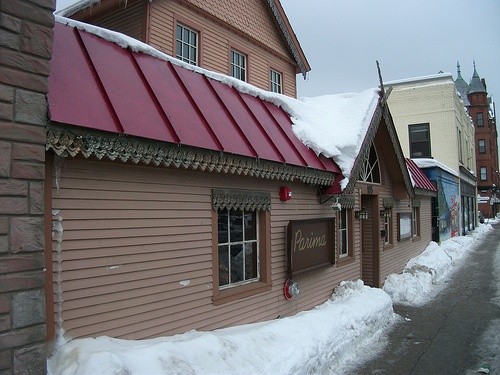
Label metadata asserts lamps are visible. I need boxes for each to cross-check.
[355,205,369,221]
[380,209,390,218]
